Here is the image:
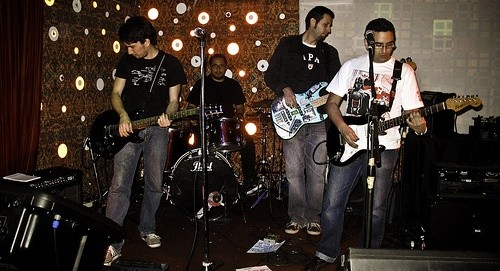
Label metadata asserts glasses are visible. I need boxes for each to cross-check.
[375,42,395,49]
[212,64,226,69]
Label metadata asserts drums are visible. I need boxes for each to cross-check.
[209,115,244,152]
[168,148,240,222]
[163,124,209,174]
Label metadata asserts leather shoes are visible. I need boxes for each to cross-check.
[304,256,328,270]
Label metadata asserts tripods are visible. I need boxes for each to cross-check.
[242,118,288,209]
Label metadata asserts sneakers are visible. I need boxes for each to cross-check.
[141,233,161,248]
[285,220,306,234]
[103,245,119,265]
[307,222,322,235]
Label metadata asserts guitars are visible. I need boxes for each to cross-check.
[270,55,418,140]
[90,103,224,158]
[325,93,483,167]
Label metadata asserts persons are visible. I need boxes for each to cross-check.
[264,5,343,235]
[102,15,187,266]
[187,53,255,186]
[304,17,427,271]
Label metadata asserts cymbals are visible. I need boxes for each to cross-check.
[249,99,274,108]
[246,112,269,119]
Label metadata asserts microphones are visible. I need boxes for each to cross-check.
[366,30,375,45]
[194,27,205,37]
[213,194,221,203]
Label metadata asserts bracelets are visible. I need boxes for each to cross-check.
[412,128,427,136]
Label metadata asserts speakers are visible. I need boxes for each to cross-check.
[336,247,500,271]
[5,166,82,227]
